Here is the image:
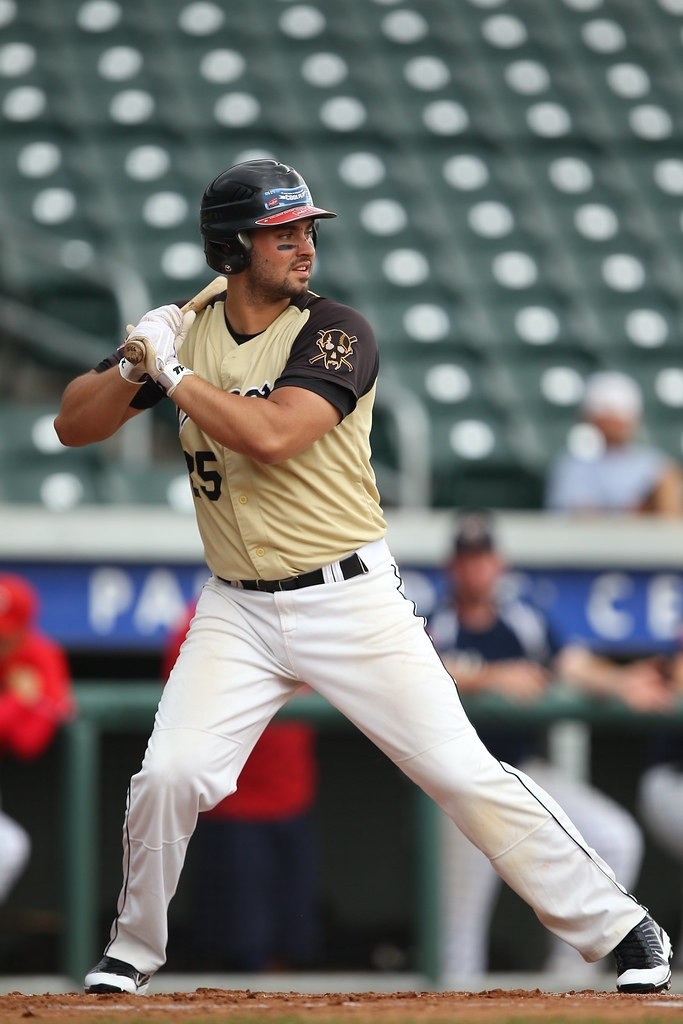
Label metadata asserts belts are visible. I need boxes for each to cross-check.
[203,552,376,594]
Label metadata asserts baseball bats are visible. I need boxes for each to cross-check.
[123,274,228,366]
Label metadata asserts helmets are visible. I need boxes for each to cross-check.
[200,158,326,282]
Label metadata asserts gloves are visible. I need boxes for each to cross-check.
[115,301,195,398]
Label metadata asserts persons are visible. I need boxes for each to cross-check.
[417,505,673,975]
[544,366,682,520]
[163,593,321,974]
[1,570,75,758]
[49,158,674,995]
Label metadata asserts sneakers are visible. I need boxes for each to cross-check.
[77,947,157,1002]
[617,905,678,996]
